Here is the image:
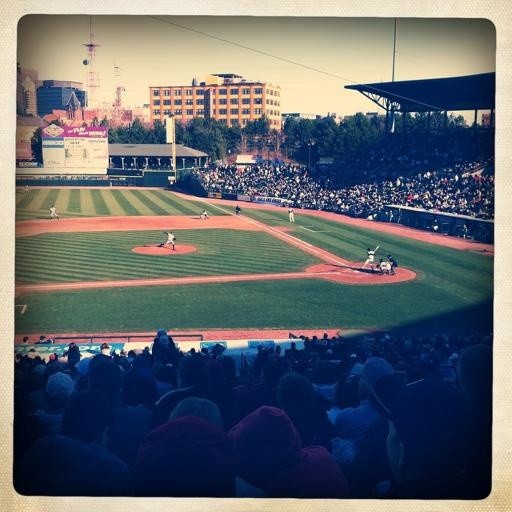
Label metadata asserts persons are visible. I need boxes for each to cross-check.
[159,231,176,250]
[358,245,379,272]
[462,224,467,239]
[388,254,398,274]
[375,257,389,275]
[15,314,494,498]
[48,205,60,221]
[193,126,492,219]
[288,206,295,223]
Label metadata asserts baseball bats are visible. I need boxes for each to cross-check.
[374,246,379,251]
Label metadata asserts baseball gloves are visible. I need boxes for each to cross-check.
[376,264,379,268]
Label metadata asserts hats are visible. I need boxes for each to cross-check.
[351,357,395,381]
[370,376,458,430]
